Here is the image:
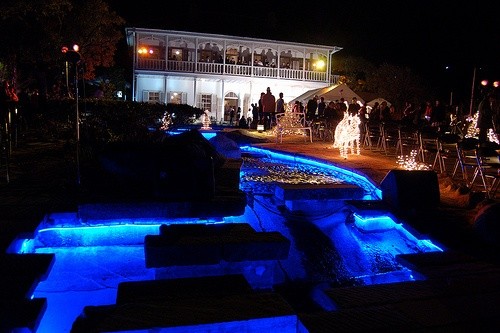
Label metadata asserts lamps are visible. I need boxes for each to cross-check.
[379,170,441,207]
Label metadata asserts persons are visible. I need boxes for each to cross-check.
[94,86,105,98]
[229,87,500,150]
[199,42,292,69]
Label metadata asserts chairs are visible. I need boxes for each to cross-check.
[315,115,500,199]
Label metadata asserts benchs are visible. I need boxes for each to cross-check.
[275,112,313,144]
